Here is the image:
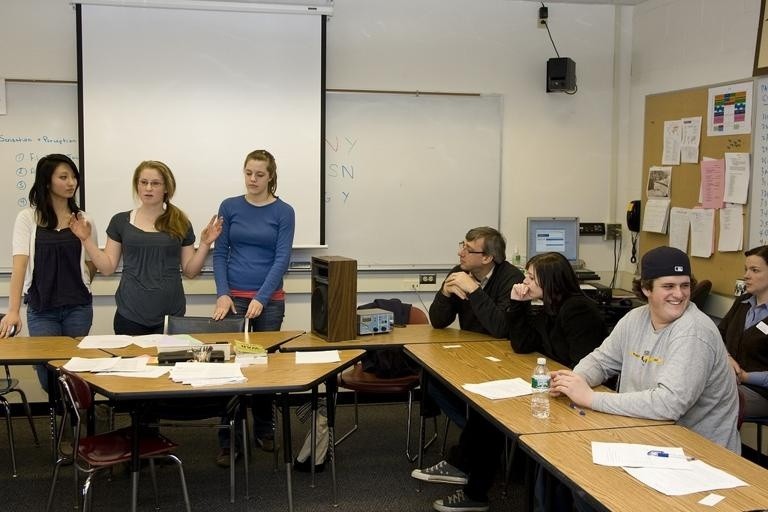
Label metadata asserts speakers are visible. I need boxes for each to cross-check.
[311,255,357,342]
[547,57,576,92]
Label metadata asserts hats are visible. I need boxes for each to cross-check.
[641,246,691,279]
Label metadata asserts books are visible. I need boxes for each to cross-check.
[232,339,266,355]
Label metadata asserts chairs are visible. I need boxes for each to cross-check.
[55,365,192,512]
[336,308,439,462]
[1,313,41,479]
[140,315,249,502]
[691,280,712,309]
[738,388,746,430]
[29,362,138,511]
[745,417,768,464]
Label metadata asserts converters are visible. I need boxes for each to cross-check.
[540,7,548,18]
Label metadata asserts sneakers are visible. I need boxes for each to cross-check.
[432,489,489,512]
[254,438,280,451]
[217,451,237,467]
[411,460,469,485]
[57,441,74,464]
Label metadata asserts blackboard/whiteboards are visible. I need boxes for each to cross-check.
[0,78,504,275]
[744,75,768,250]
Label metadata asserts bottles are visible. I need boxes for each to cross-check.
[529,357,551,421]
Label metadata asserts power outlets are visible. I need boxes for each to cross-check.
[538,19,548,28]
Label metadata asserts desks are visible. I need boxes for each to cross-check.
[404,327,677,512]
[1,323,507,459]
[516,424,768,512]
[46,349,368,512]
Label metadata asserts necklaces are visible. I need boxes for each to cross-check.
[56,209,66,232]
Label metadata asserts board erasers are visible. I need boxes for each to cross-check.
[290,262,311,269]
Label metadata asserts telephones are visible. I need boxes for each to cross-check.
[627,200,641,233]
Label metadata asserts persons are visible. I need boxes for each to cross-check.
[428,226,526,339]
[717,245,768,419]
[547,246,743,459]
[212,150,295,467]
[0,154,98,461]
[68,160,224,472]
[411,251,614,512]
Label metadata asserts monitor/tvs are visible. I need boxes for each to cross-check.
[520,217,580,267]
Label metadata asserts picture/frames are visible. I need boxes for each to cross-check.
[753,0,768,76]
[604,223,622,241]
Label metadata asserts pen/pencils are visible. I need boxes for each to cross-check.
[648,450,696,460]
[204,341,230,344]
[568,402,586,415]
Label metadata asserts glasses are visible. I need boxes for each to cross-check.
[459,241,485,253]
[138,180,165,187]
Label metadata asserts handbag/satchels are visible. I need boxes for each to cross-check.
[294,407,329,473]
